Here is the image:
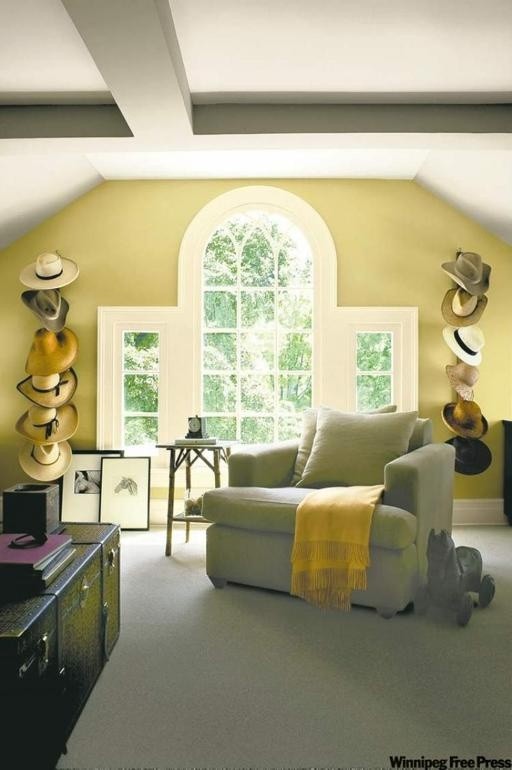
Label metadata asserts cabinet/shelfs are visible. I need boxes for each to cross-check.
[0,522,123,770]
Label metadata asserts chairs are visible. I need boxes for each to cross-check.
[203,408,458,619]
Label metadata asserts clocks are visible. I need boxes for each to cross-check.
[186,416,211,440]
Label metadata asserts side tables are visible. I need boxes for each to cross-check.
[153,441,237,555]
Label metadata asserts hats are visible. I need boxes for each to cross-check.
[14,404,79,446]
[18,439,73,483]
[440,252,491,298]
[17,368,78,409]
[21,288,71,333]
[445,435,492,475]
[20,251,79,291]
[440,401,488,440]
[440,289,489,328]
[25,326,79,377]
[442,323,484,367]
[445,362,480,402]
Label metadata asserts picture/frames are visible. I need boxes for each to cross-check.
[60,449,150,531]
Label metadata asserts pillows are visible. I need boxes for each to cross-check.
[290,405,418,487]
